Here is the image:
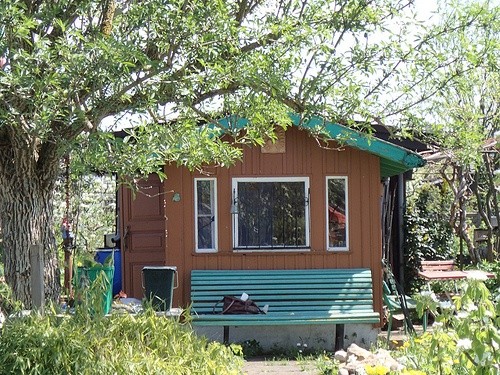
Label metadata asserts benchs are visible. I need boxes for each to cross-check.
[188,268,380,355]
[420,260,458,293]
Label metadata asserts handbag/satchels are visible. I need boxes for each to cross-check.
[212,296,266,315]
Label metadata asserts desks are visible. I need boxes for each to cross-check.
[420,270,495,295]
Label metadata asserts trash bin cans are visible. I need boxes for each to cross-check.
[73,264,116,316]
[96,248,121,297]
[140,266,180,312]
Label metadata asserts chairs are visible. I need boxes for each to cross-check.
[381,279,427,343]
